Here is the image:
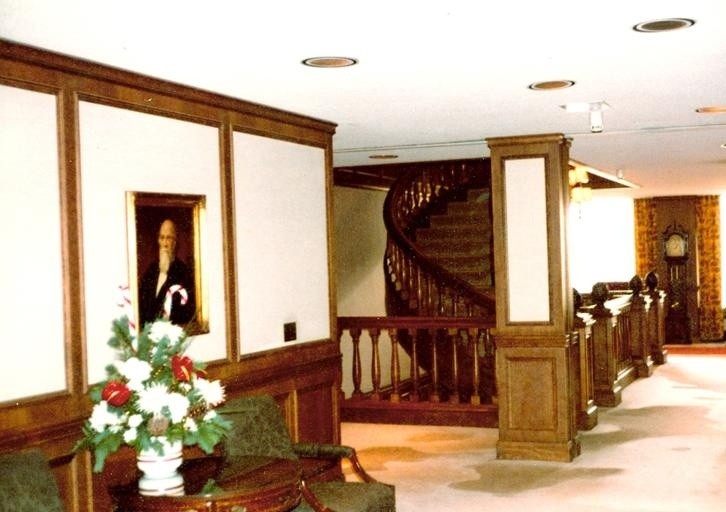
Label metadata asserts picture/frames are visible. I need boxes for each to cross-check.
[124,190,210,342]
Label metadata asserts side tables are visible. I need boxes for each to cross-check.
[125,455,304,511]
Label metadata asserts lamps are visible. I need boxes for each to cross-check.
[568,168,593,205]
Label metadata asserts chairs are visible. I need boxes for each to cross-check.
[1,449,65,512]
[218,394,396,511]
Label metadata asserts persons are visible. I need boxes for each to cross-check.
[139,219,196,332]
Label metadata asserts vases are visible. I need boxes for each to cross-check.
[133,438,184,496]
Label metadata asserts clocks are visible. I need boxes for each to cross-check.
[662,218,693,344]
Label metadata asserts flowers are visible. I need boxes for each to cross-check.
[70,315,233,472]
